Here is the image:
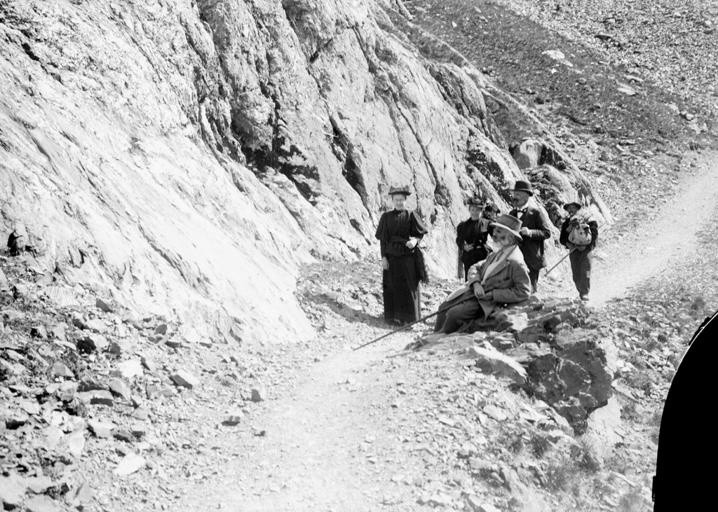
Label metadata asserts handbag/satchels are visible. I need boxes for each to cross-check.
[537,255,547,268]
[414,242,431,282]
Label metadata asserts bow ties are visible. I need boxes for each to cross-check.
[516,209,525,214]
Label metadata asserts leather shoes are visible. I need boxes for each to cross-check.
[581,293,589,301]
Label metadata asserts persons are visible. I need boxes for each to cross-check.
[434,214,530,334]
[456,199,495,282]
[509,181,553,292]
[375,186,429,323]
[559,200,598,300]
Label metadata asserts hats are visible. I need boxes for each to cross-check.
[388,185,411,195]
[467,196,484,208]
[489,214,524,241]
[563,202,582,210]
[509,181,533,197]
[485,203,501,213]
